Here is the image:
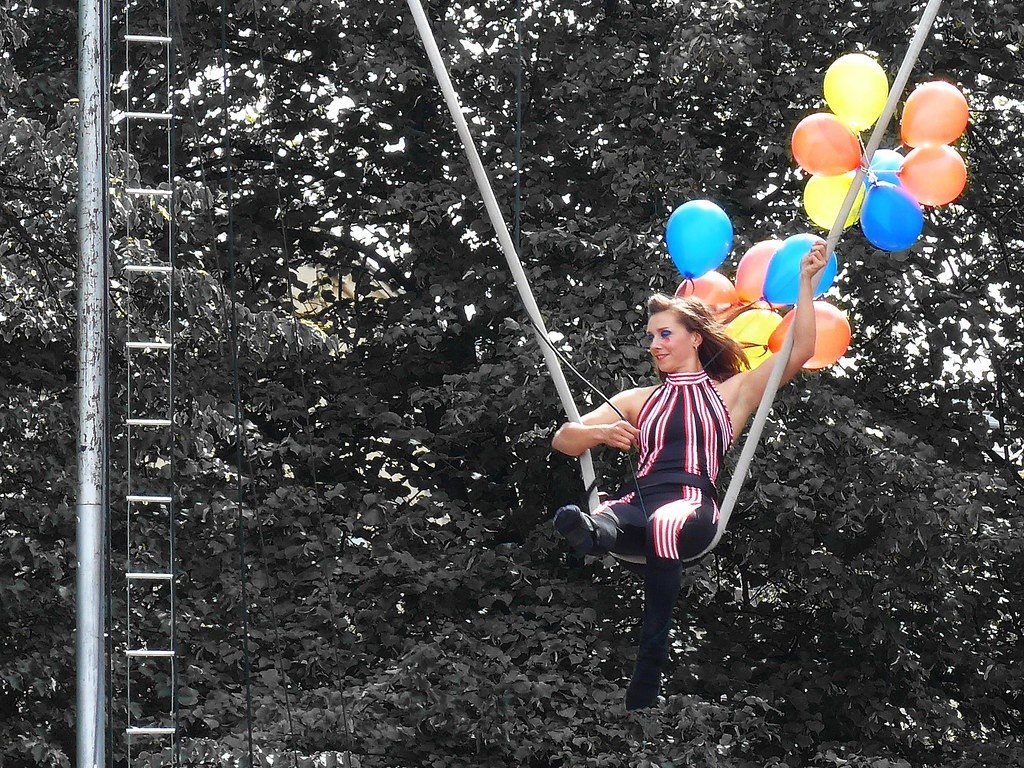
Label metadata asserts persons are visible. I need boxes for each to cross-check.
[551,238,828,712]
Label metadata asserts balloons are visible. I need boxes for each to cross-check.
[667,52,968,370]
